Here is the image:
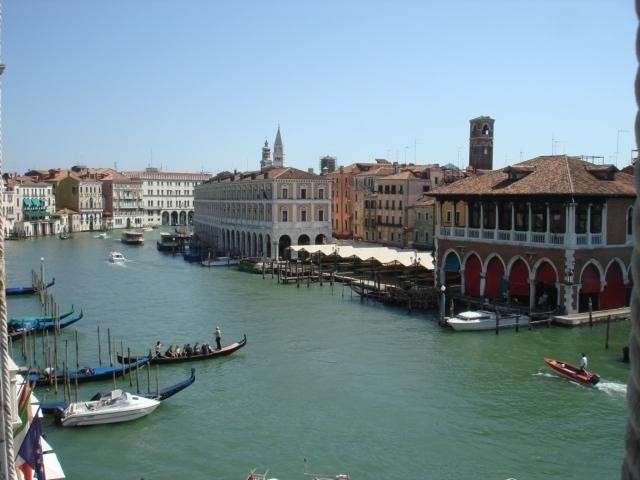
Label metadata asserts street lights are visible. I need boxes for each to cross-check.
[439,283,447,320]
[39,257,46,285]
[410,249,422,286]
[272,239,279,263]
[5,277,58,297]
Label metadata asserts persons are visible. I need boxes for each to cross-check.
[212,326,221,350]
[580,352,587,375]
[155,340,210,357]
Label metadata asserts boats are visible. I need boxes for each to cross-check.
[155,232,179,252]
[199,256,238,267]
[174,228,191,234]
[60,233,73,241]
[540,356,601,387]
[121,230,145,245]
[446,308,531,332]
[108,250,125,264]
[8,302,248,429]
[239,256,270,274]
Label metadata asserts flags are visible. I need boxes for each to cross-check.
[11,388,35,480]
[19,412,46,480]
[18,380,34,424]
